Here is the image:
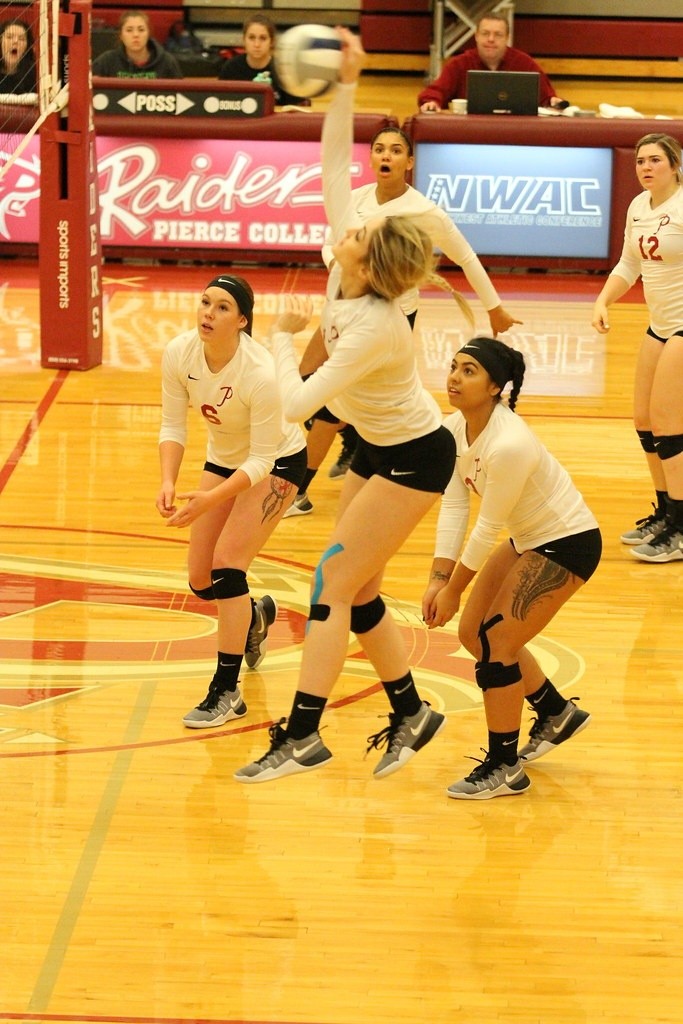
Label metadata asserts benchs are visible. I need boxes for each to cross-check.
[0,0,184,43]
[360,0,682,62]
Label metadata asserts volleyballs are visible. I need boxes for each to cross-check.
[274,23,344,102]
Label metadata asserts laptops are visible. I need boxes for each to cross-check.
[466,69,540,117]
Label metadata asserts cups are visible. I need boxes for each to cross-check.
[452,98,468,115]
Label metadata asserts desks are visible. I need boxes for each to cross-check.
[0,102,41,256]
[401,112,683,269]
[92,114,400,261]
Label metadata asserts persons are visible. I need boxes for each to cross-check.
[591,132,683,562]
[416,11,566,113]
[280,121,526,530]
[216,14,308,107]
[0,18,38,95]
[232,25,456,786]
[167,19,204,54]
[155,273,308,731]
[421,333,602,800]
[88,11,185,80]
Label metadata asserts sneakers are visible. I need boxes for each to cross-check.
[328,440,357,480]
[368,701,444,779]
[182,681,247,728]
[619,502,667,544]
[630,523,683,562]
[447,748,531,799]
[519,696,592,763]
[282,492,314,517]
[232,718,335,783]
[245,596,278,669]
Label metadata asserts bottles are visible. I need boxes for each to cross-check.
[252,72,272,85]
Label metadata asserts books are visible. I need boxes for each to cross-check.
[537,106,578,118]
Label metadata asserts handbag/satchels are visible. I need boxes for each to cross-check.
[163,20,204,54]
[176,48,226,76]
